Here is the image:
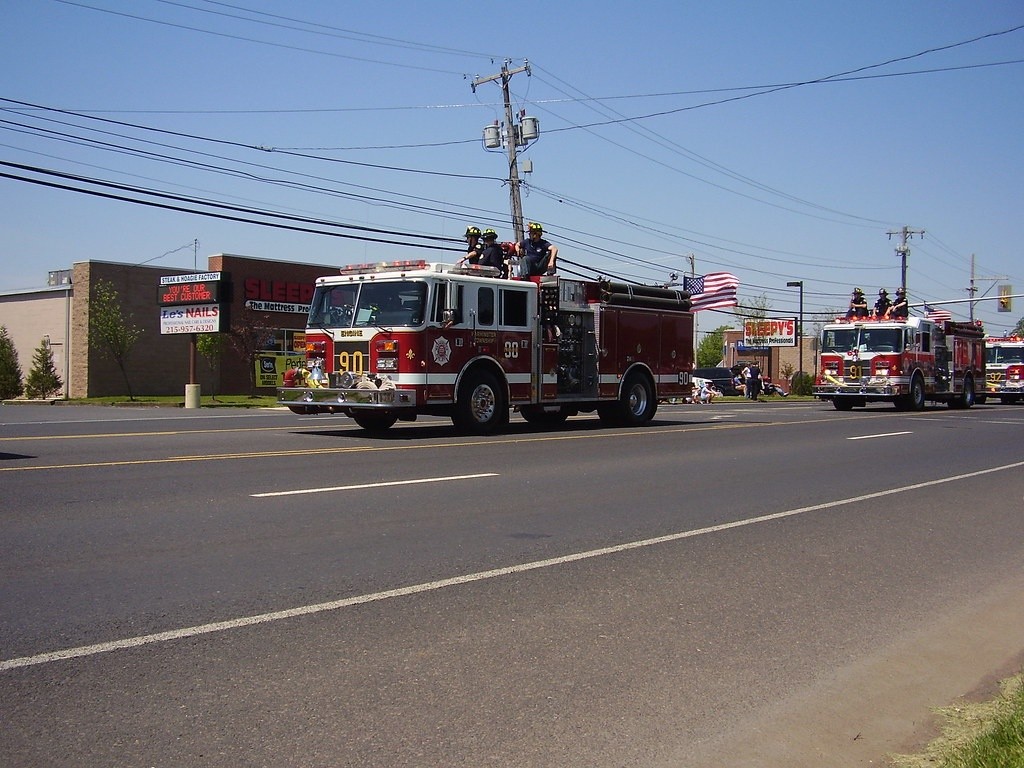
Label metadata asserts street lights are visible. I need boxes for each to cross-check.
[787,281,803,396]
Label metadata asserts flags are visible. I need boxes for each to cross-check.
[924,305,951,323]
[682,271,739,312]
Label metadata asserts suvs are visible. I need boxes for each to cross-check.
[693,363,745,397]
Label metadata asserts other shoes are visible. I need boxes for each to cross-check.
[520,275,530,281]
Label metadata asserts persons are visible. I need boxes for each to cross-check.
[672,382,719,404]
[870,288,909,319]
[456,226,509,279]
[734,360,789,401]
[515,222,559,282]
[845,286,869,319]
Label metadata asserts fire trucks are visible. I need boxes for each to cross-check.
[276,259,693,438]
[982,329,1024,405]
[811,316,987,413]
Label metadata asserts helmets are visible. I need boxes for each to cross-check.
[463,227,481,238]
[878,288,889,295]
[482,229,497,239]
[526,223,548,233]
[852,287,865,295]
[895,288,907,295]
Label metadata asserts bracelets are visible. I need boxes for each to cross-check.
[463,257,467,260]
